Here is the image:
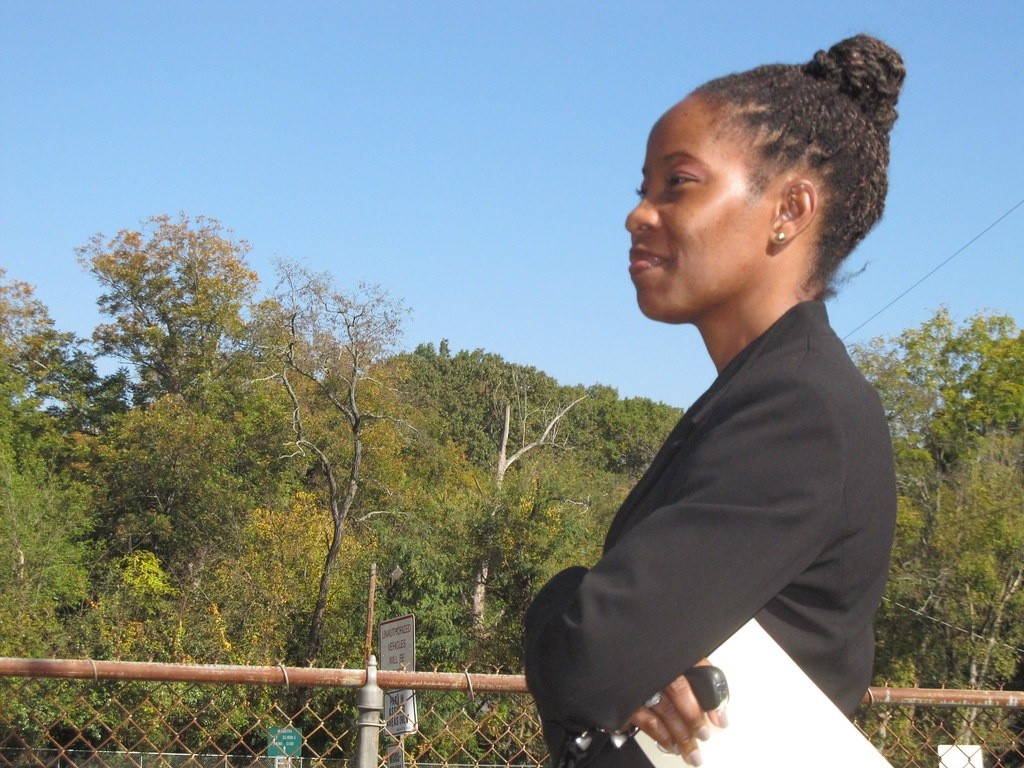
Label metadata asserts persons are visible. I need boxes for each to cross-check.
[524,36,907,768]
